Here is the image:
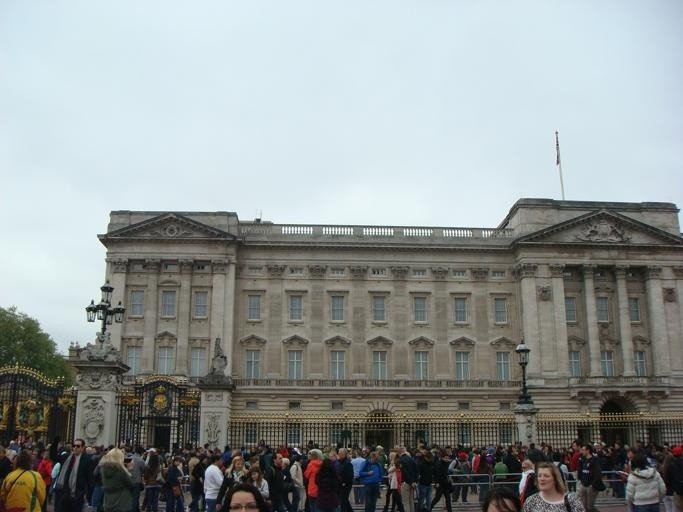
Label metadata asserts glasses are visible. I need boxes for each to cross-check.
[227,500,259,512]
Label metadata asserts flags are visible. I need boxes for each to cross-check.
[555,134,559,165]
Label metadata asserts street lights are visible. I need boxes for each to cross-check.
[514,337,534,403]
[85,280,125,335]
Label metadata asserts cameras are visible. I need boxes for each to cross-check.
[123,458,133,463]
